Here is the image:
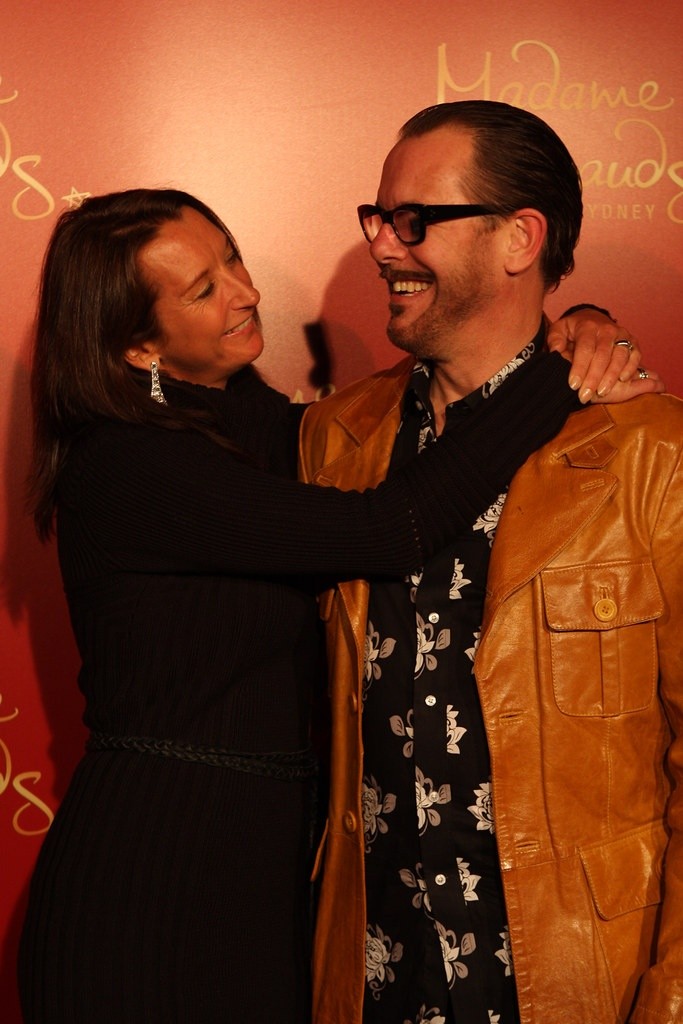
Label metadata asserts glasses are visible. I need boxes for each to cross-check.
[355,203,497,246]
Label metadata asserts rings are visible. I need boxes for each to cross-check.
[637,367,649,380]
[615,340,633,351]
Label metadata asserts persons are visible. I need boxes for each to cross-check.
[293,99,682,1024]
[18,189,665,1024]
